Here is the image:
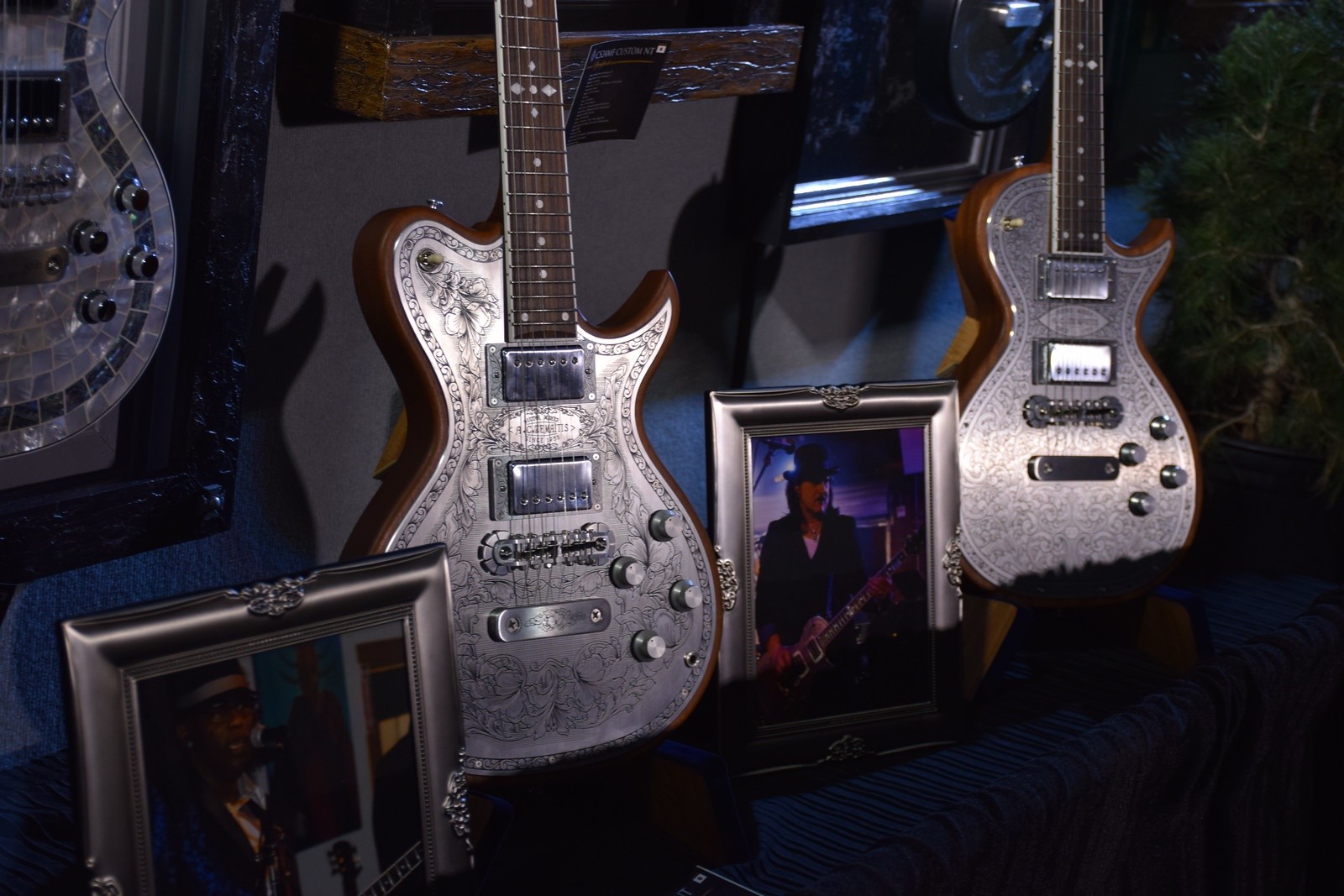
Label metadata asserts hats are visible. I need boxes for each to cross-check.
[172,658,259,717]
[783,444,840,480]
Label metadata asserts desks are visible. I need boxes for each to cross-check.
[0,559,1344,896]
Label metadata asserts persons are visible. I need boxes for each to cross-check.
[153,661,314,896]
[751,446,925,724]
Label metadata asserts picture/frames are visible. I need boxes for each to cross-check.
[708,378,965,780]
[765,0,1015,247]
[58,543,476,896]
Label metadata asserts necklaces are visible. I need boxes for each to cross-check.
[806,521,821,542]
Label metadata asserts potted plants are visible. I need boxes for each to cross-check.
[1125,0,1344,570]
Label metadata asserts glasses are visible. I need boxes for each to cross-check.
[199,702,258,721]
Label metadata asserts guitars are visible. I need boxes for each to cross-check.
[0,0,180,461]
[753,530,922,712]
[335,0,725,788]
[950,0,1206,603]
